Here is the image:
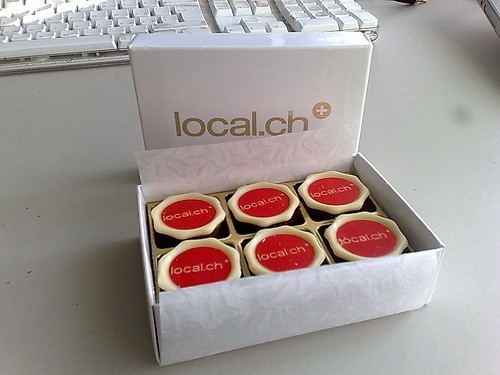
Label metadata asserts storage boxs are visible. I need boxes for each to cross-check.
[126,31,445,367]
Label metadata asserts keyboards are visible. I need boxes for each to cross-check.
[0,0,378,76]
